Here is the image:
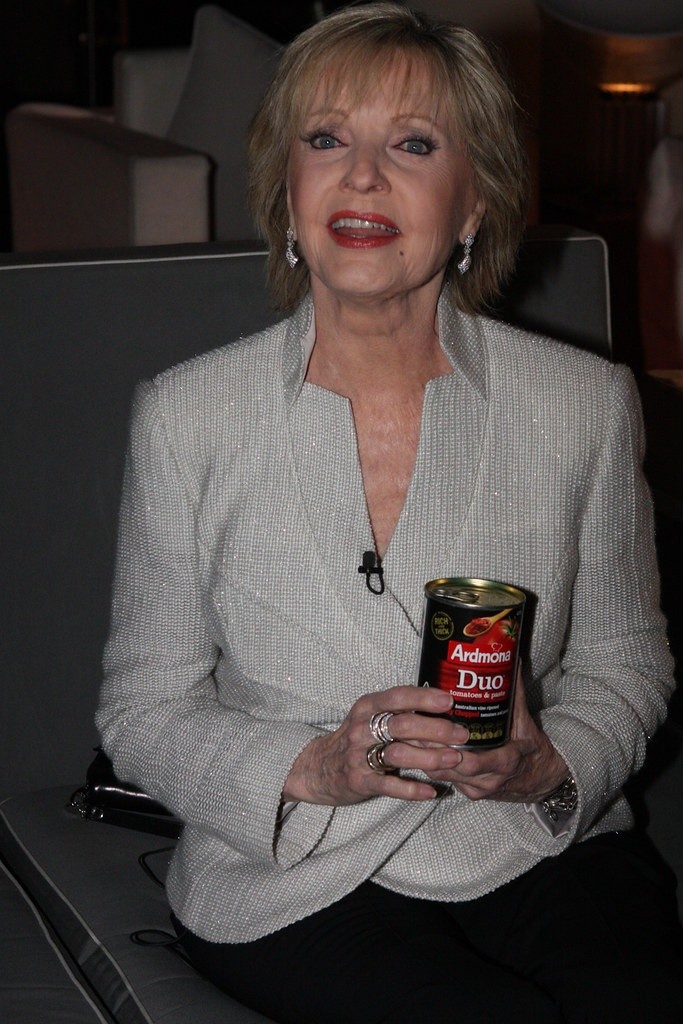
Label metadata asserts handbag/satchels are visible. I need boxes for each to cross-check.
[66,746,183,842]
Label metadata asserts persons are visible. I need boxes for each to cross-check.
[92,2,683,1023]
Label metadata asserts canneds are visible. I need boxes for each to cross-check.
[410,576,527,750]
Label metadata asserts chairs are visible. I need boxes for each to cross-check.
[0,10,683,1024]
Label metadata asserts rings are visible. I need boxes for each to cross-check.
[369,711,394,771]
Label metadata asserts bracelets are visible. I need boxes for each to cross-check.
[545,773,577,822]
